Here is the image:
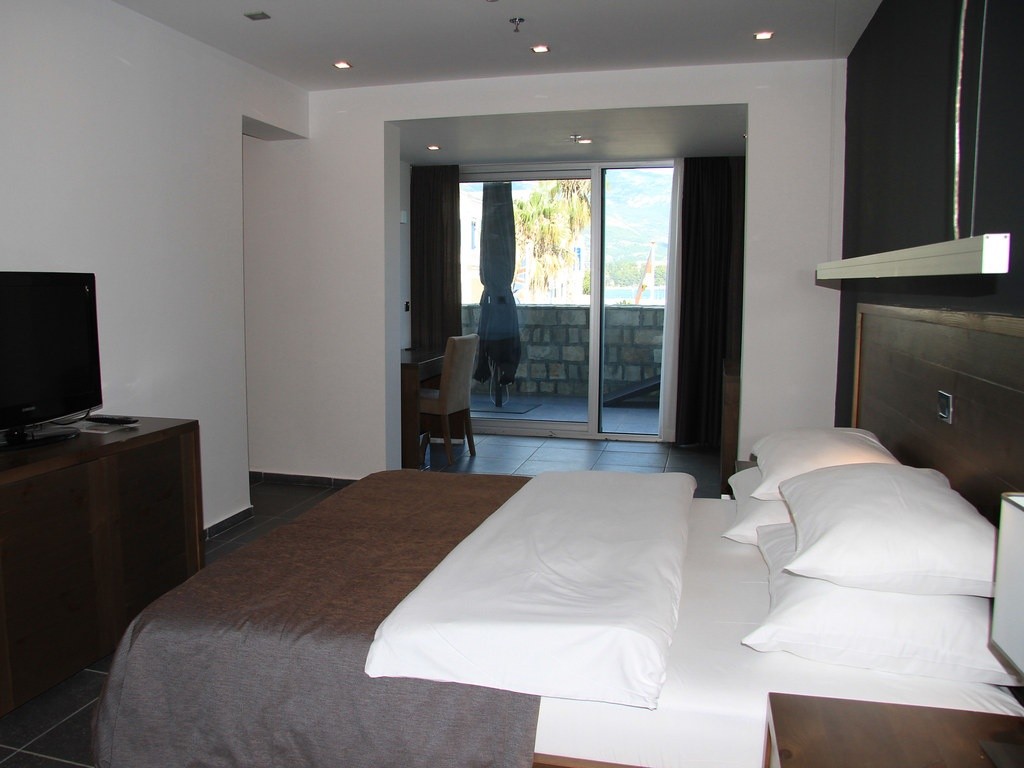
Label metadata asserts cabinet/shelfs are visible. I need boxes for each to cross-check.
[720,366,740,480]
[0,413,206,719]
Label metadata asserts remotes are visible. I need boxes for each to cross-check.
[86,414,139,424]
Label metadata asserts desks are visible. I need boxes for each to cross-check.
[401,347,447,469]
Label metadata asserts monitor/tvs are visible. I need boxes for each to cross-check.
[0,270,103,453]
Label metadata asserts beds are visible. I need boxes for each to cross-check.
[86,302,1024,768]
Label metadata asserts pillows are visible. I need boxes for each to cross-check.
[721,466,795,546]
[751,427,901,501]
[737,521,1021,687]
[779,462,996,598]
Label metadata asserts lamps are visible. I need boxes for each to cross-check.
[816,232,1010,281]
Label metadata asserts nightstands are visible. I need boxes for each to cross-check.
[760,692,1024,768]
[731,461,758,476]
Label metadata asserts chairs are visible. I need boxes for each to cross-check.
[419,334,481,463]
